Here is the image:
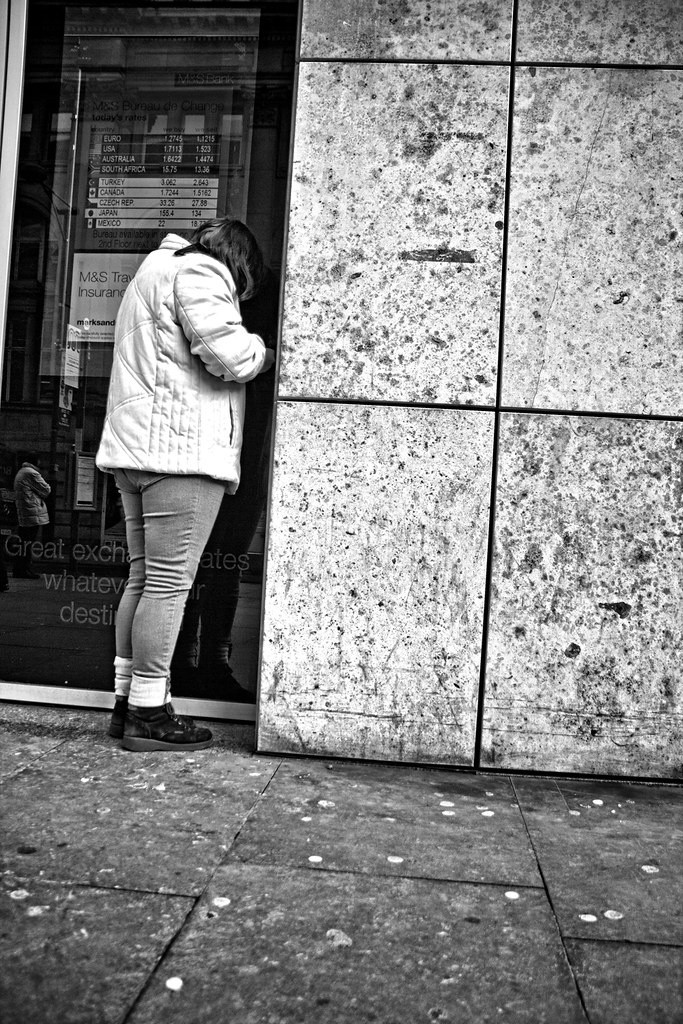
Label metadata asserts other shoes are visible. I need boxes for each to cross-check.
[0,583,10,590]
[12,568,40,579]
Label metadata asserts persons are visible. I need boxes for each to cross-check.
[14,450,51,579]
[96,218,275,748]
[167,262,276,704]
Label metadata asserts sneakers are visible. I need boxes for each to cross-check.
[122,701,214,750]
[167,665,256,703]
[108,694,130,740]
